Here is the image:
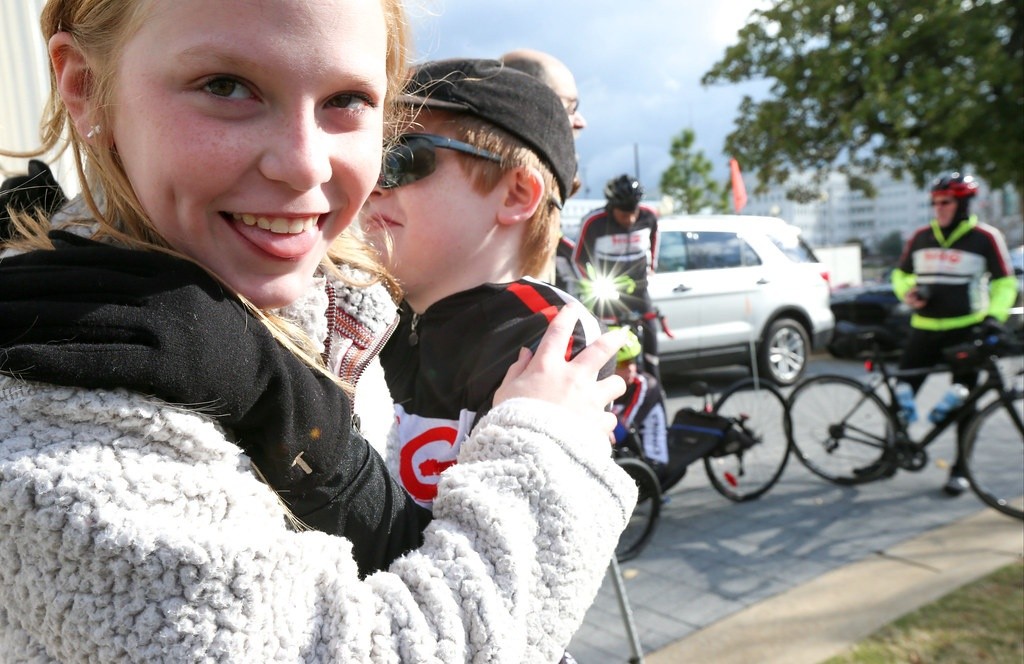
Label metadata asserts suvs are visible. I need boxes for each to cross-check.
[632,216,834,387]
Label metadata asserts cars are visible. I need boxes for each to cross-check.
[829,284,1024,356]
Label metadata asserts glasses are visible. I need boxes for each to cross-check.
[564,98,578,115]
[932,200,954,207]
[380,133,565,213]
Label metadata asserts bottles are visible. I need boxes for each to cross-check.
[895,379,919,424]
[929,383,969,426]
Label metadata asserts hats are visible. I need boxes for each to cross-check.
[394,60,578,200]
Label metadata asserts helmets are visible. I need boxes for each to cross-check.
[932,172,977,198]
[604,175,644,210]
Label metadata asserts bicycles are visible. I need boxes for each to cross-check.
[596,306,793,503]
[588,419,665,567]
[782,326,1024,526]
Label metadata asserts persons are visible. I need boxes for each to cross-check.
[843,170,1018,495]
[350,56,575,574]
[599,322,666,474]
[0,0,637,663]
[493,46,583,142]
[578,177,661,394]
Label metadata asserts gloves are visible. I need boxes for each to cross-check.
[980,316,1006,343]
[0,229,354,495]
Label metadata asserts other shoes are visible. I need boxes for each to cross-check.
[853,462,897,479]
[946,470,969,492]
